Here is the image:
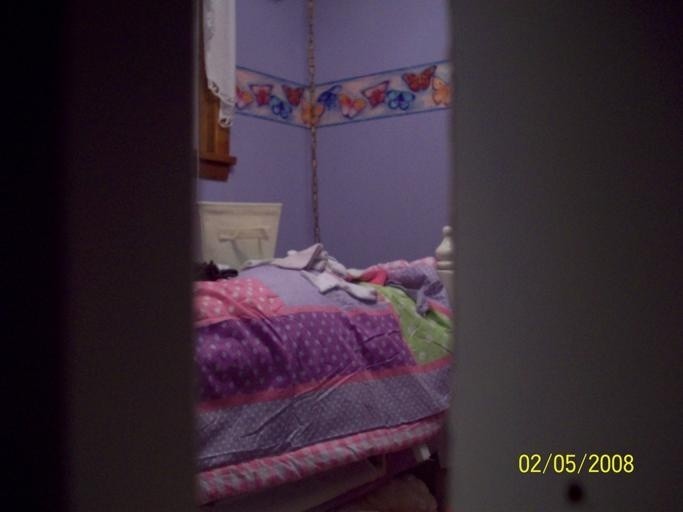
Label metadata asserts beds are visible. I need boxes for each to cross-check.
[194,225,453,508]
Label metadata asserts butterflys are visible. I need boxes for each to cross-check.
[235,64,451,126]
